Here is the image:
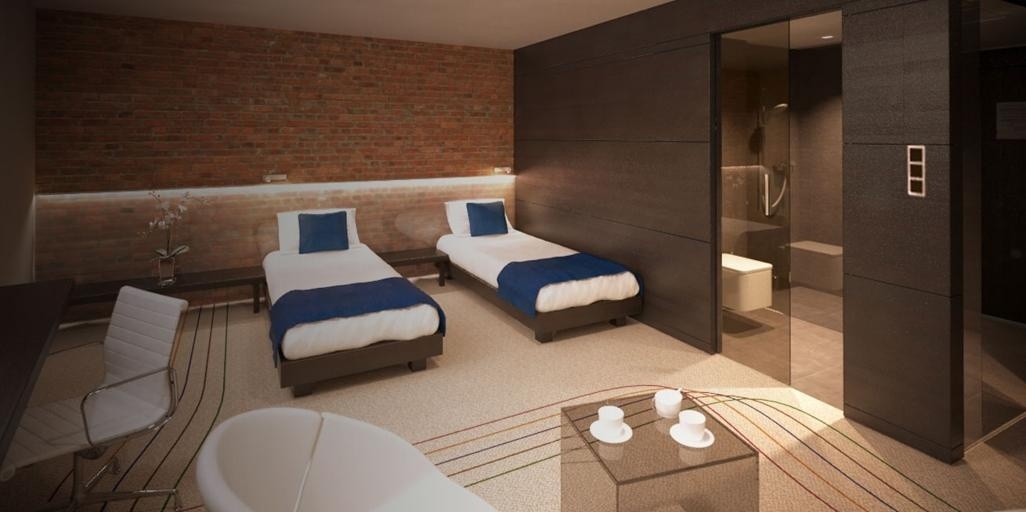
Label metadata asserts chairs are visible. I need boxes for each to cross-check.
[0,286,191,511]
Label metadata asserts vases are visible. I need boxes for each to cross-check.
[156,255,176,287]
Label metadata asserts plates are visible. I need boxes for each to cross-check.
[670,423,715,448]
[590,419,633,444]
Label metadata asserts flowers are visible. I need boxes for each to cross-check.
[132,190,212,263]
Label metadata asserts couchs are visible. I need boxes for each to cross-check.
[192,406,497,511]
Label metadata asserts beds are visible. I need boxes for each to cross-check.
[262,207,445,397]
[434,197,643,344]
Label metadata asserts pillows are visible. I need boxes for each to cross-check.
[276,208,360,255]
[444,198,514,236]
[466,201,508,236]
[298,211,350,254]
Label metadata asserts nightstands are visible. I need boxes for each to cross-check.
[376,248,448,286]
[64,265,266,313]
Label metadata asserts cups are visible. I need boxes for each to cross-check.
[651,389,682,419]
[656,415,679,435]
[679,409,706,444]
[679,444,704,466]
[598,406,625,438]
[597,441,625,461]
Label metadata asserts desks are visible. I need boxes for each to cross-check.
[0,279,71,473]
